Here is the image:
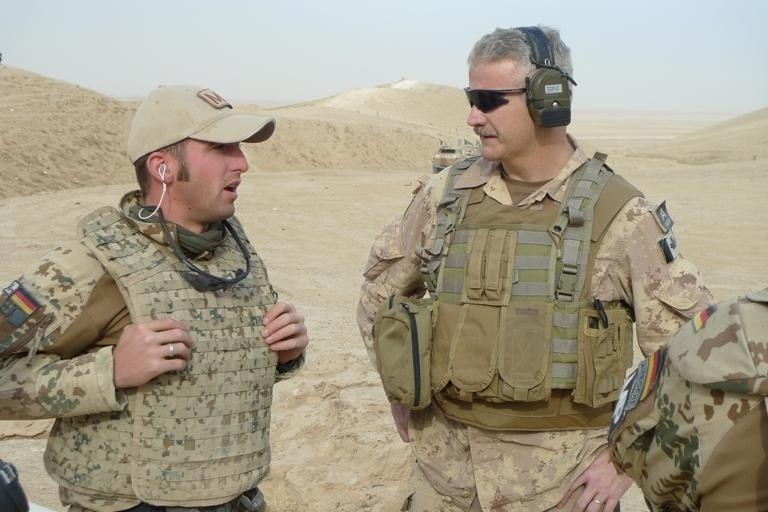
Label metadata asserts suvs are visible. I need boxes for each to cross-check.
[432,144,481,174]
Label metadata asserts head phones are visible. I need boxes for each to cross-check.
[514,26,571,128]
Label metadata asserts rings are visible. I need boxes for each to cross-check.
[594,500,601,504]
[168,343,174,357]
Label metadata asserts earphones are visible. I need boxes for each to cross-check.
[158,163,167,186]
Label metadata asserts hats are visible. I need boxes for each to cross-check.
[127,84,276,165]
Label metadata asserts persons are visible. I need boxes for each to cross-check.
[355,26,716,512]
[0,83,309,511]
[607,288,768,512]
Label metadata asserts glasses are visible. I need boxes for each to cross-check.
[463,86,526,114]
[157,207,252,292]
